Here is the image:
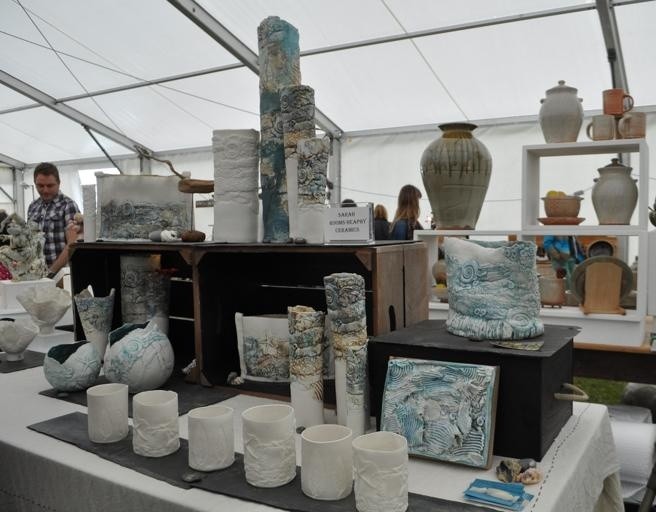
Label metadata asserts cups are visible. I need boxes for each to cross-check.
[351,430,409,512]
[241,403,298,489]
[132,389,181,459]
[187,405,235,472]
[602,88,634,119]
[617,111,646,139]
[586,114,615,141]
[86,383,129,444]
[300,423,353,502]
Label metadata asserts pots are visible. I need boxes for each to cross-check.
[541,196,585,217]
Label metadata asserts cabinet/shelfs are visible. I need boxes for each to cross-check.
[414,137,648,324]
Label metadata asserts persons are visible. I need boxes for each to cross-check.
[0,209,10,246]
[372,203,390,240]
[541,237,586,306]
[388,184,425,239]
[25,161,80,278]
[341,199,358,208]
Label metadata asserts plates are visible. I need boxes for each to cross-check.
[536,217,586,225]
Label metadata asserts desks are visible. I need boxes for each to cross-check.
[1,328,609,512]
